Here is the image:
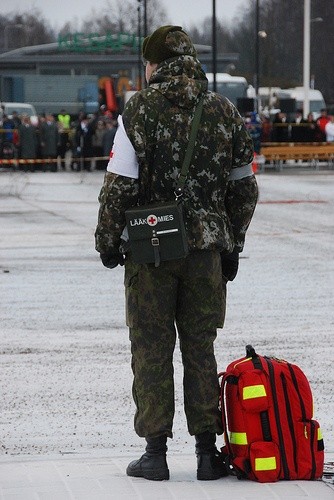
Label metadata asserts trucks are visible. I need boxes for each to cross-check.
[0,101,39,128]
[205,72,328,123]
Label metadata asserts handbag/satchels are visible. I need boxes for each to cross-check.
[125,200,189,267]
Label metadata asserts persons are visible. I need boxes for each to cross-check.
[241,109,334,155]
[0,105,118,170]
[95,25,259,480]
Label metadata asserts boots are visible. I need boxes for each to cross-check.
[195,433,227,480]
[126,437,170,480]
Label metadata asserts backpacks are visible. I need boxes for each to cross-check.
[216,344,324,484]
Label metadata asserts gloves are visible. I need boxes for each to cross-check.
[221,252,239,280]
[99,251,125,269]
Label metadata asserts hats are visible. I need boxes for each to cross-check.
[143,25,196,62]
